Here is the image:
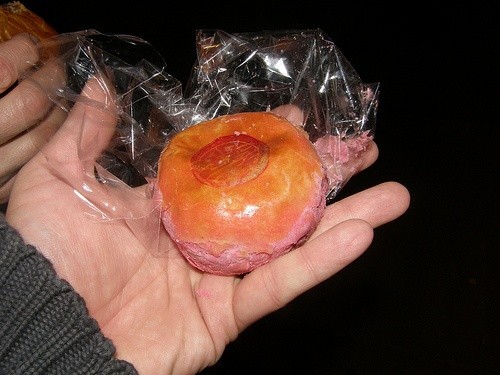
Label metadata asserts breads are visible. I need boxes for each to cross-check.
[0,1,61,68]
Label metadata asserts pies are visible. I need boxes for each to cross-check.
[156,111,327,276]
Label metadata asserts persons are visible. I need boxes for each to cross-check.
[0,63,412,374]
